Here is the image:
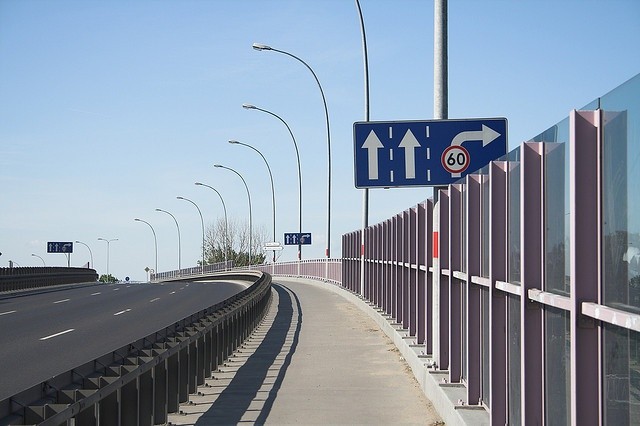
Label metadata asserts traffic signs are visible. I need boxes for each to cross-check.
[440,146,470,173]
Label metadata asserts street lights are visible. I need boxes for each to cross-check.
[241,103,302,275]
[134,219,157,280]
[176,196,204,274]
[194,181,228,271]
[76,240,94,268]
[97,238,119,282]
[155,208,181,275]
[228,138,276,273]
[214,163,252,270]
[31,253,45,266]
[252,42,332,278]
